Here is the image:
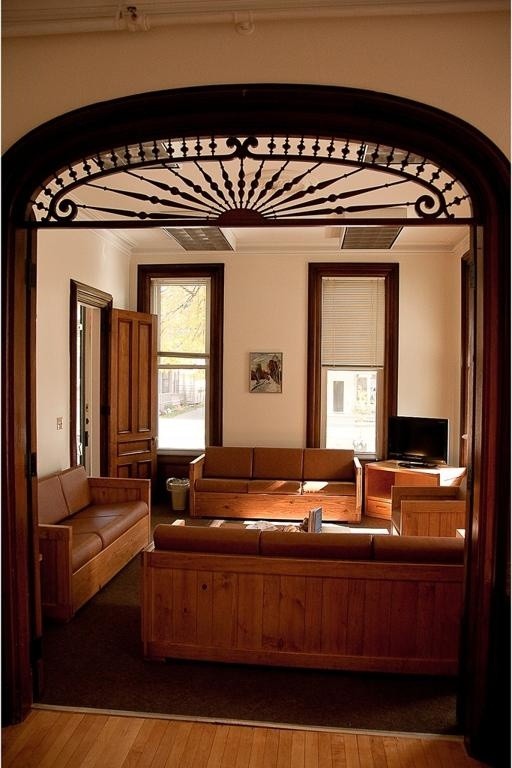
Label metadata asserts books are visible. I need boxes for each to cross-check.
[313,505,323,534]
[308,508,317,533]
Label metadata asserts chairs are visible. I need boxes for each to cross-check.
[389,469,471,537]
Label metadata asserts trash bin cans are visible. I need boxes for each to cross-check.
[168,479,190,510]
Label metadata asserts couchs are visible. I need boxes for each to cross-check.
[139,520,468,676]
[37,465,152,624]
[188,446,363,524]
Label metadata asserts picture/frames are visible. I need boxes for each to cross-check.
[250,352,283,393]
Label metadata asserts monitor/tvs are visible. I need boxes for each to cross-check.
[387,416,449,469]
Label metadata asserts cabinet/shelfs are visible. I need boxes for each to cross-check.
[364,459,446,521]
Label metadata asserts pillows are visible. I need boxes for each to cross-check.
[202,446,354,481]
[37,465,93,524]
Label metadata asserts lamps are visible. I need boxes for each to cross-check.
[340,207,407,249]
[361,143,431,165]
[96,141,182,171]
[160,210,238,252]
